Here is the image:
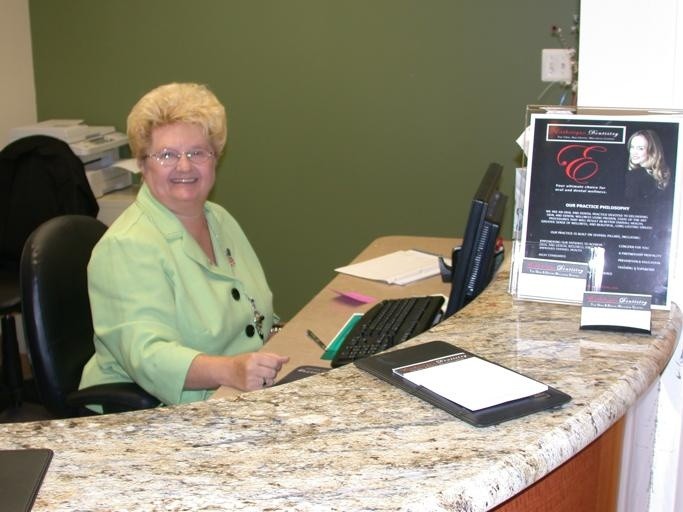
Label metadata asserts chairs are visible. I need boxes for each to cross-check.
[1,134,100,414]
[19,213,161,417]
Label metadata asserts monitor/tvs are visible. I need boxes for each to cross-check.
[444,162,508,317]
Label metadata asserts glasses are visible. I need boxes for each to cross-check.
[144,148,215,166]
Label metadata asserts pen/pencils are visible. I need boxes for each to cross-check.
[306,328,326,351]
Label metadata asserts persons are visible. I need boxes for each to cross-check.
[621,129,671,204]
[71,79,291,420]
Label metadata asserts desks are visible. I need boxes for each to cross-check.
[207,233,514,399]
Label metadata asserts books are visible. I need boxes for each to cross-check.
[349,337,573,430]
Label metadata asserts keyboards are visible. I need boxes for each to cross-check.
[331,296,444,367]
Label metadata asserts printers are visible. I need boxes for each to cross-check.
[11,117,131,198]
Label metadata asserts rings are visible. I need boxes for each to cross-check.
[262,378,266,386]
